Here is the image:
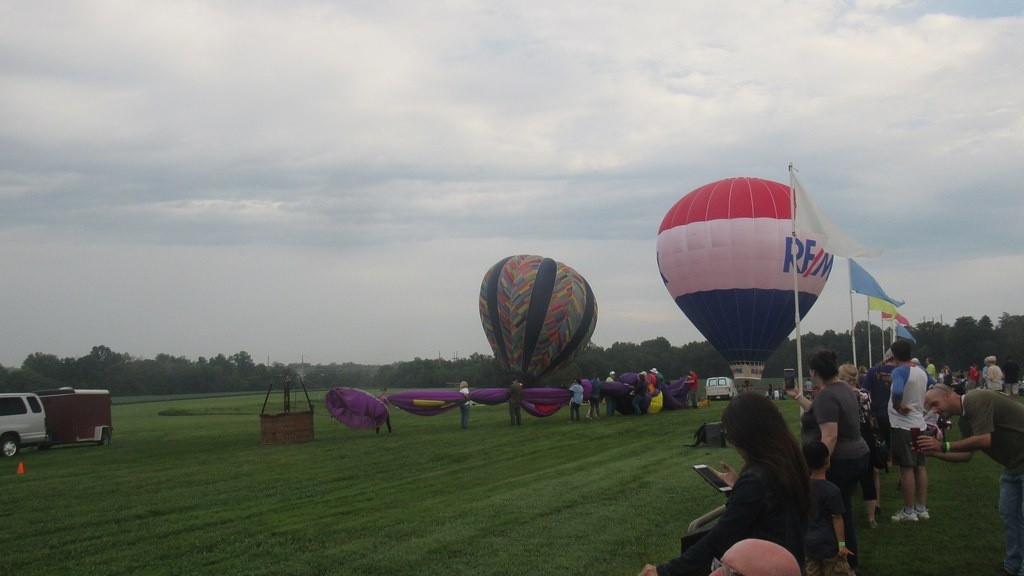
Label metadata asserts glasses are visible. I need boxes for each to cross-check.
[711,558,744,576]
[928,383,947,394]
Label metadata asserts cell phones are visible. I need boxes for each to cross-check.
[911,427,923,450]
[784,369,795,389]
[693,464,732,493]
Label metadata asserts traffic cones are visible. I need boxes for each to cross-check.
[16,462,25,474]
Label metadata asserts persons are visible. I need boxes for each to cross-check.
[767,383,772,400]
[804,379,820,399]
[586,374,603,420]
[1002,356,1020,399]
[836,347,902,529]
[636,391,814,576]
[630,373,648,416]
[459,381,469,428]
[802,441,857,576]
[684,369,698,408]
[925,358,980,395]
[982,356,1004,391]
[887,340,936,521]
[569,378,584,422]
[509,376,522,426]
[373,386,392,434]
[647,367,658,385]
[910,383,1024,576]
[783,345,870,570]
[605,371,616,417]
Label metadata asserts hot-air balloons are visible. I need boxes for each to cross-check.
[656,176,834,397]
[478,254,599,390]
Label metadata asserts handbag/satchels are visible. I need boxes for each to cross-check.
[862,425,890,468]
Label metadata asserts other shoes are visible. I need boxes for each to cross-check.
[868,520,878,529]
[875,505,880,512]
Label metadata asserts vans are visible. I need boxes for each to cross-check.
[0,392,52,459]
[705,377,737,400]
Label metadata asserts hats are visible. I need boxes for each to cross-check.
[911,357,920,365]
[650,367,659,374]
[609,371,615,374]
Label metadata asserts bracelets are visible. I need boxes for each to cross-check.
[795,393,801,399]
[838,542,845,546]
[946,442,950,452]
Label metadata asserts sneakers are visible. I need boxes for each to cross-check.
[890,506,919,520]
[914,505,929,520]
